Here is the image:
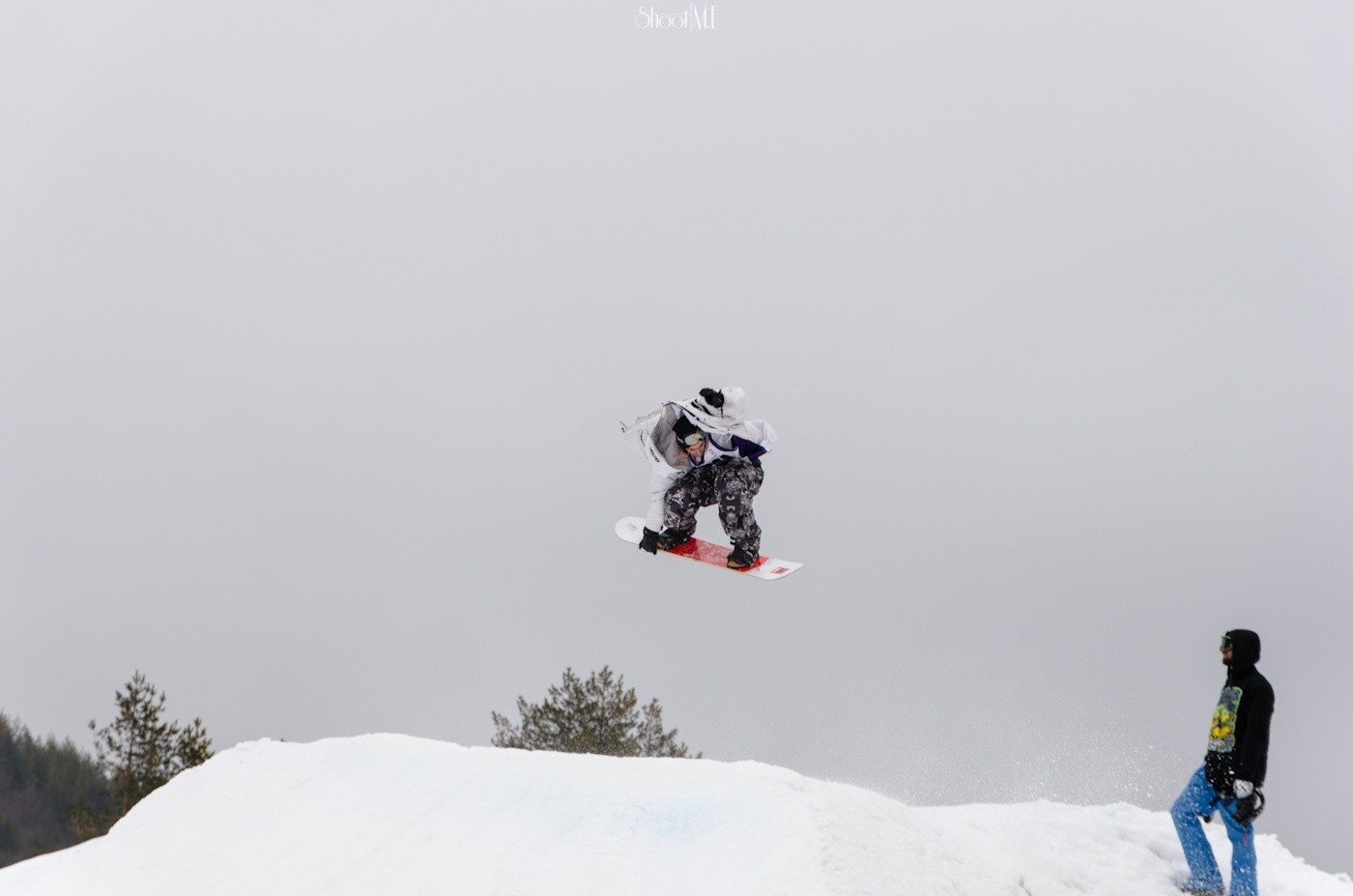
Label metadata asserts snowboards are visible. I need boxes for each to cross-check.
[615,516,805,581]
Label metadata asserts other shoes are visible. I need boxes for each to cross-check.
[1182,886,1223,896]
[727,546,758,568]
[655,528,690,549]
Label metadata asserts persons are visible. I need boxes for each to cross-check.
[1170,628,1275,896]
[639,388,775,568]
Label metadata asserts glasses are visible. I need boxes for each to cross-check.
[1219,635,1233,652]
[684,429,705,446]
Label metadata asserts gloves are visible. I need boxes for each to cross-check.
[1233,780,1254,800]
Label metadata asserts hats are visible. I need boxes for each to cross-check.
[673,416,700,447]
